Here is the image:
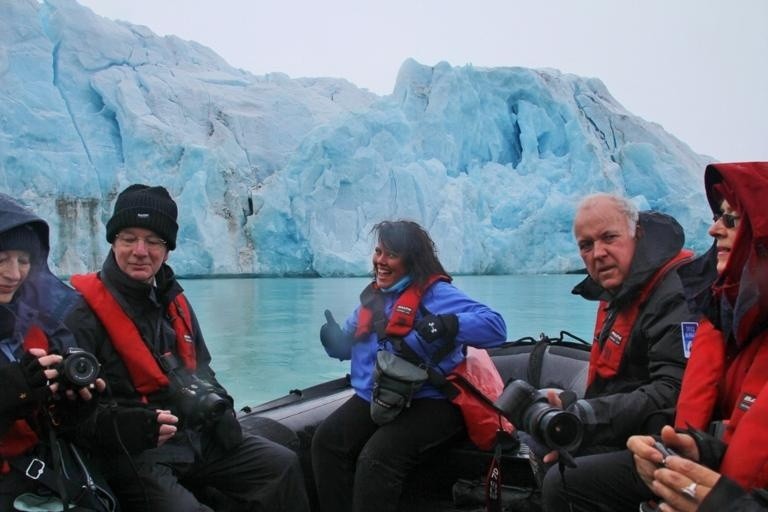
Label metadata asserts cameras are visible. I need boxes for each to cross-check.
[171,381,228,425]
[45,346,102,389]
[493,380,584,454]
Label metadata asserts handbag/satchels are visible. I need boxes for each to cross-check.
[370,350,429,427]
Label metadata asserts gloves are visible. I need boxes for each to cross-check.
[56,384,107,425]
[695,473,768,511]
[319,309,340,345]
[647,426,729,471]
[0,349,49,414]
[102,406,163,454]
[414,302,447,342]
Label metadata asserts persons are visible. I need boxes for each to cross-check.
[309,218,508,510]
[540,162,767,512]
[539,191,707,512]
[624,340,767,512]
[0,190,117,511]
[64,182,317,510]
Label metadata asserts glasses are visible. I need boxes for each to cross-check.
[713,211,740,228]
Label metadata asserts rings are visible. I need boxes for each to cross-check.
[679,482,698,500]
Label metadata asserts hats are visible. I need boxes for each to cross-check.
[0,227,40,255]
[106,184,177,251]
